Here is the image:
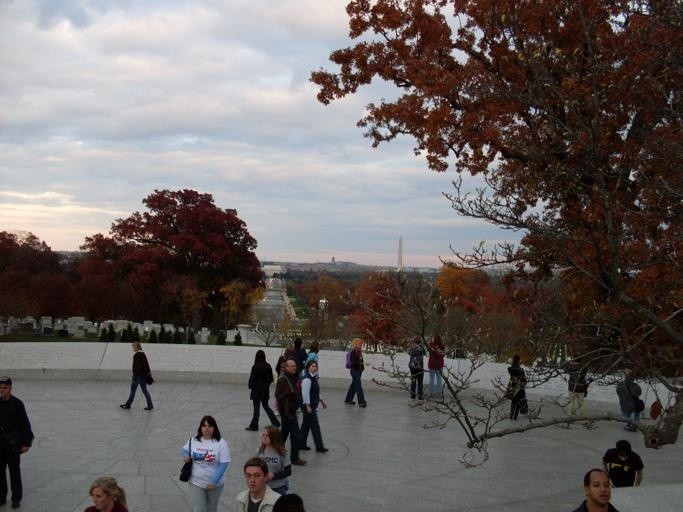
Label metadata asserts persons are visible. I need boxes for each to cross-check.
[118,339,155,412]
[572,468,619,512]
[559,356,588,425]
[251,424,293,495]
[404,336,426,399]
[614,372,643,433]
[0,372,34,509]
[233,458,281,512]
[343,336,367,407]
[80,475,130,511]
[427,333,446,399]
[245,337,330,466]
[271,494,303,511]
[183,414,231,511]
[603,440,644,487]
[506,354,526,421]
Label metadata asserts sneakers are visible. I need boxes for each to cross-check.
[292,459,305,466]
[317,448,328,453]
[120,404,130,409]
[245,427,258,431]
[144,405,153,410]
[345,401,355,404]
[298,446,310,451]
[360,403,367,407]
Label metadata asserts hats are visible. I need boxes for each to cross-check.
[0,376,12,385]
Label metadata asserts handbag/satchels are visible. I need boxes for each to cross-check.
[520,398,530,415]
[635,400,645,412]
[180,459,193,482]
[346,352,352,369]
